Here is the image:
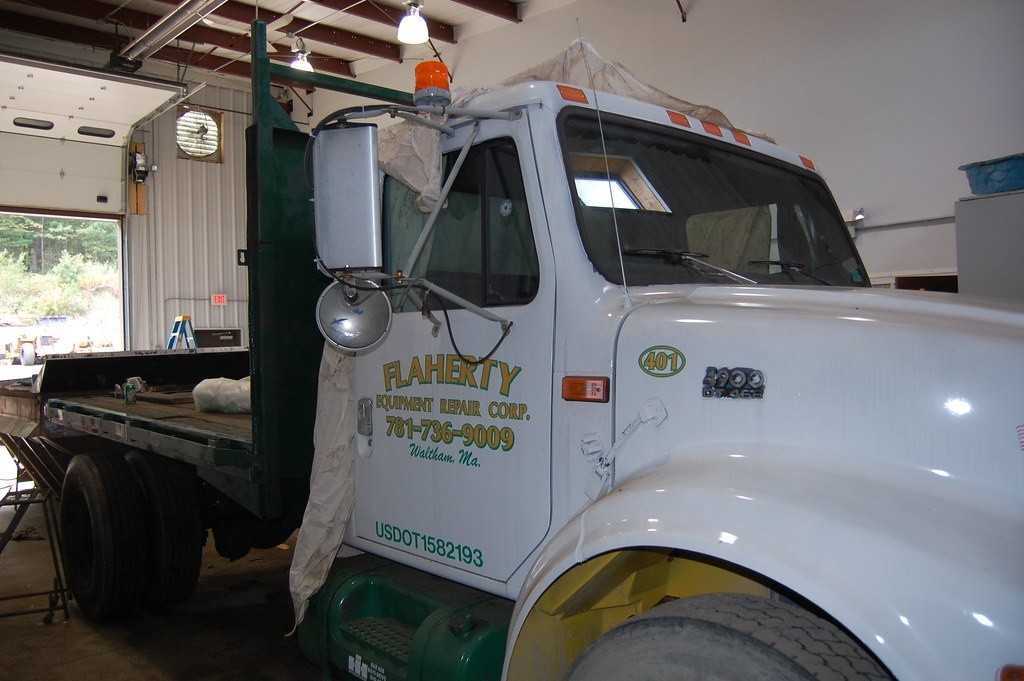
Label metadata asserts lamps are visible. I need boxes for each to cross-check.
[290,38,315,72]
[397,0,430,46]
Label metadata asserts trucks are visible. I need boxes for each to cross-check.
[0,16,1020,680]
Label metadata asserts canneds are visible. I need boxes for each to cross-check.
[124,384,136,405]
